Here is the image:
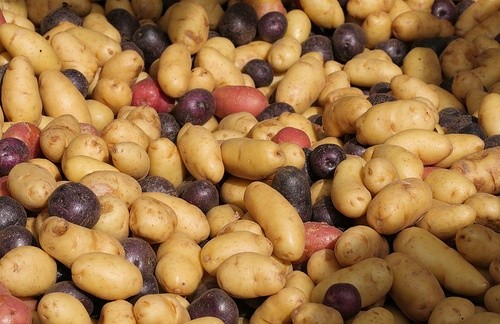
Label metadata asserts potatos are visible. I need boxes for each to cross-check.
[0,0,500,324]
